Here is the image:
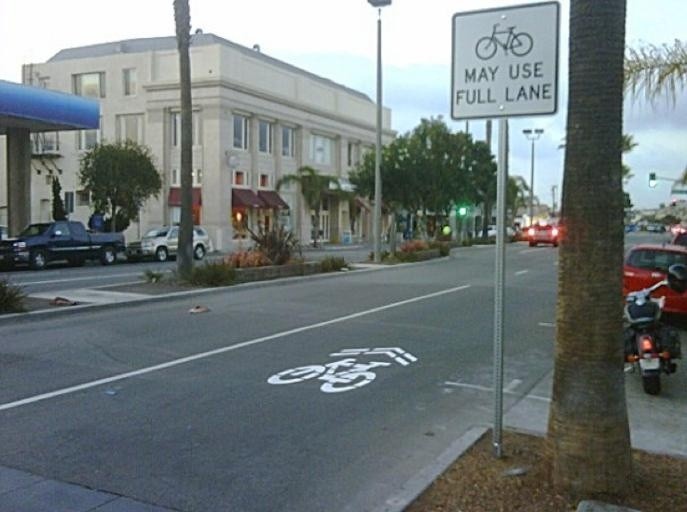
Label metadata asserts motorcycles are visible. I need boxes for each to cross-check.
[621,280,683,397]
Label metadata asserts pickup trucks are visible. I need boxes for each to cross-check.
[0,222,126,271]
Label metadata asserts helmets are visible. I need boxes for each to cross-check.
[668,263,687,292]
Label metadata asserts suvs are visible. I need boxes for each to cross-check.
[125,226,211,262]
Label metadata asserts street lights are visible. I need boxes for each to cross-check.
[523,129,544,226]
[366,0,392,263]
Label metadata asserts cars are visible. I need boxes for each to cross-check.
[0,224,8,239]
[622,224,687,328]
[476,224,559,248]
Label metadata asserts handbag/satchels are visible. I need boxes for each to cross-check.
[624,327,639,363]
[655,324,682,360]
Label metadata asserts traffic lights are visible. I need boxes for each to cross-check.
[456,206,468,218]
